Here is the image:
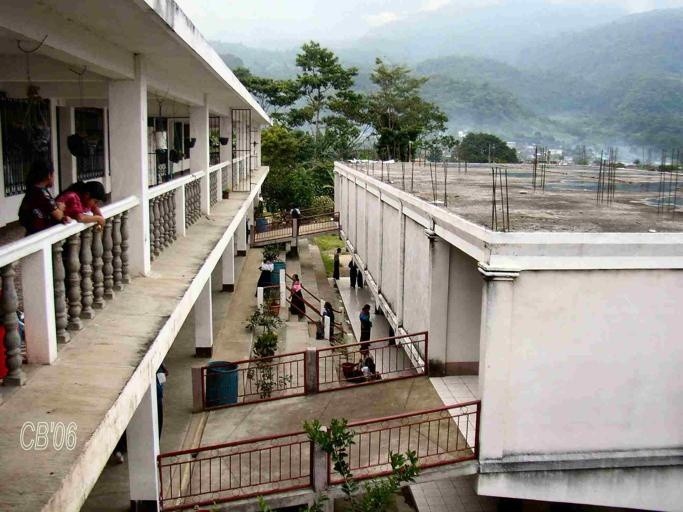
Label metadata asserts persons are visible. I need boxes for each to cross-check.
[156,362,169,436]
[290,204,302,236]
[288,274,305,317]
[324,302,334,335]
[254,207,260,221]
[18,158,71,235]
[256,255,275,298]
[114,433,126,465]
[334,249,375,382]
[54,181,106,225]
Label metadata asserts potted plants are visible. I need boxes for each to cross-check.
[222,186,231,199]
[243,303,282,336]
[265,291,280,317]
[169,150,184,163]
[67,133,100,157]
[331,333,354,377]
[253,329,278,363]
[14,119,50,150]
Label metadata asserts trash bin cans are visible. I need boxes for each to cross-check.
[205,361,239,408]
[256,218,267,233]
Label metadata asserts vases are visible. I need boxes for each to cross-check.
[219,138,228,145]
[186,138,196,149]
